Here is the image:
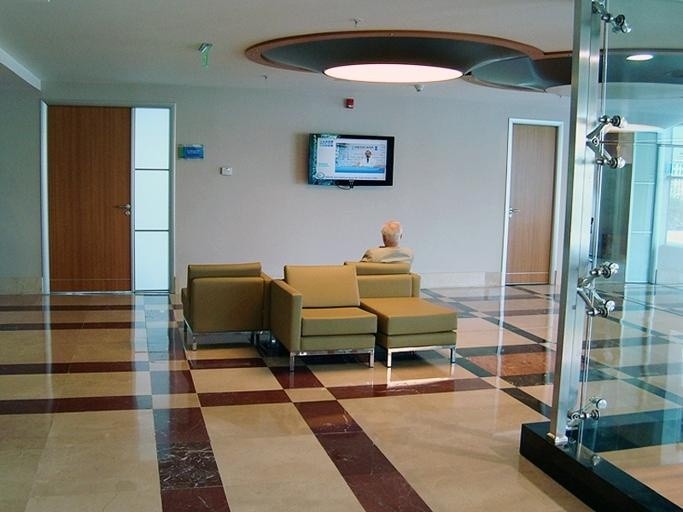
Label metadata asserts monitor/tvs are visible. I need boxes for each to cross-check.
[308,133,395,187]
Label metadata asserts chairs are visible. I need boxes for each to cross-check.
[181,259,457,371]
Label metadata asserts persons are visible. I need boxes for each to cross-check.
[361,220,414,262]
[358,150,375,167]
